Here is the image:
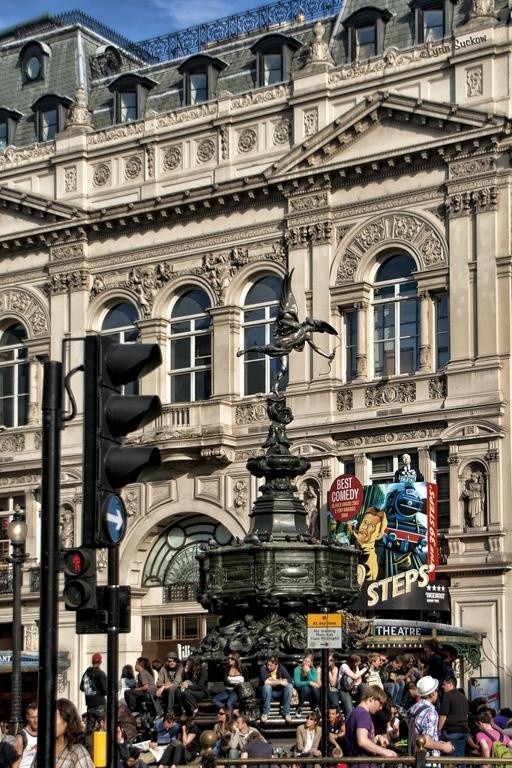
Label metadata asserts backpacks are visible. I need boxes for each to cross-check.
[482,739,512,768]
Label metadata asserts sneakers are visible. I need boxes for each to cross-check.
[168,705,383,723]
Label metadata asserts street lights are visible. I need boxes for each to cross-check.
[3,504,31,738]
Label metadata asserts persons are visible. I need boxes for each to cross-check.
[79,653,108,721]
[229,715,266,758]
[240,731,272,768]
[359,652,424,709]
[327,705,346,767]
[423,638,454,684]
[54,699,95,767]
[463,470,485,526]
[317,651,340,707]
[438,675,470,768]
[293,653,321,717]
[294,712,323,767]
[85,712,129,768]
[212,652,250,711]
[466,707,511,768]
[406,676,454,768]
[11,702,38,767]
[116,652,209,768]
[235,318,336,399]
[347,507,387,588]
[12,504,26,522]
[257,656,293,723]
[60,511,74,548]
[344,684,397,767]
[337,654,370,715]
[394,451,424,483]
[212,707,237,758]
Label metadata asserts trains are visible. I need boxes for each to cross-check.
[380,483,429,553]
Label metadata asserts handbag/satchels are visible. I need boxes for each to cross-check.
[338,673,355,693]
[236,679,256,700]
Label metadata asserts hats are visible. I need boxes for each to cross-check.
[91,652,102,663]
[166,651,178,659]
[415,675,440,697]
[0,741,22,768]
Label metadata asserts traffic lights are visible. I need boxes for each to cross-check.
[60,551,92,610]
[106,344,165,486]
